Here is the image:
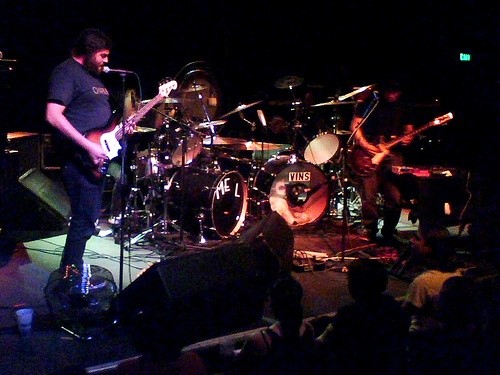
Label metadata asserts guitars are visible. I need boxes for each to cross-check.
[76,77,178,178]
[346,112,455,180]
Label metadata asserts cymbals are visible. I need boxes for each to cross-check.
[339,83,375,101]
[194,118,279,152]
[218,98,265,118]
[132,124,157,132]
[310,96,363,107]
[139,95,197,106]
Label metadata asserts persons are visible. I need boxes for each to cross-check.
[226,256,500,375]
[44,28,137,267]
[116,304,207,375]
[350,69,416,244]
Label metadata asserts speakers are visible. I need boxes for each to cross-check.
[109,239,293,346]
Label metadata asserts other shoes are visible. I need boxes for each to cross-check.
[380,226,401,241]
[59,267,83,280]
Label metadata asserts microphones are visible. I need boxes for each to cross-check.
[102,64,134,72]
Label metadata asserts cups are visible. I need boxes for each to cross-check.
[16,308,33,334]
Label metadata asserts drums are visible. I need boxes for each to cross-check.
[136,149,164,176]
[247,154,331,231]
[165,161,249,242]
[295,126,342,165]
